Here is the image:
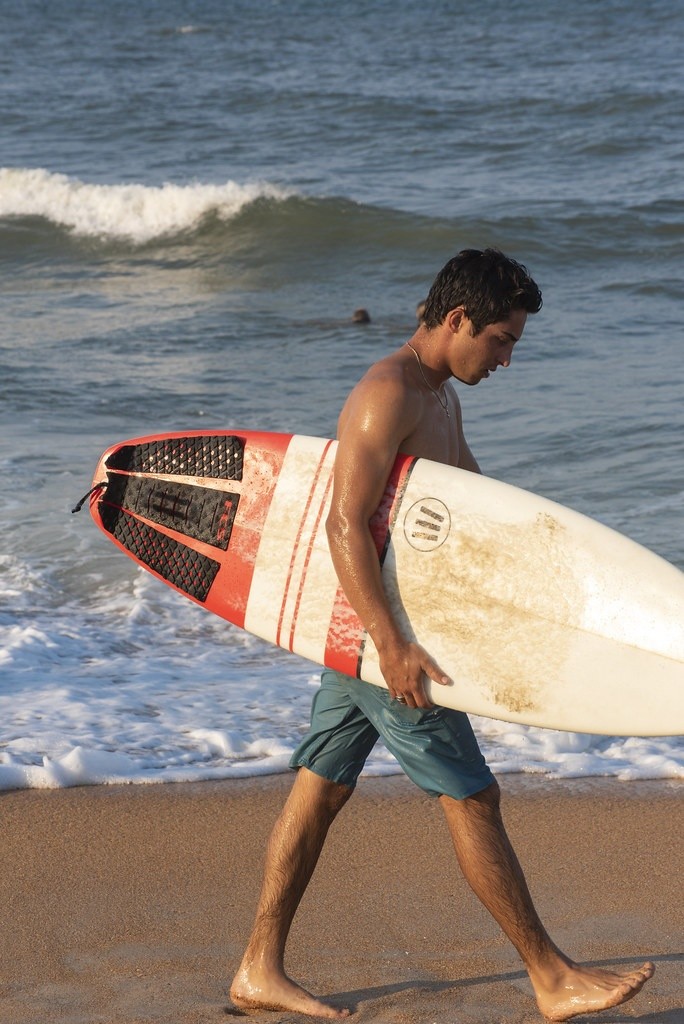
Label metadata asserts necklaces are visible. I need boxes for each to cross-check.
[406,341,450,418]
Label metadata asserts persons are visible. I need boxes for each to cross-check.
[229,245,655,1021]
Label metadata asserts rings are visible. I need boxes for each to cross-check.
[396,697,404,702]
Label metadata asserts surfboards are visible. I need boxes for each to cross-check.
[88,429,684,736]
